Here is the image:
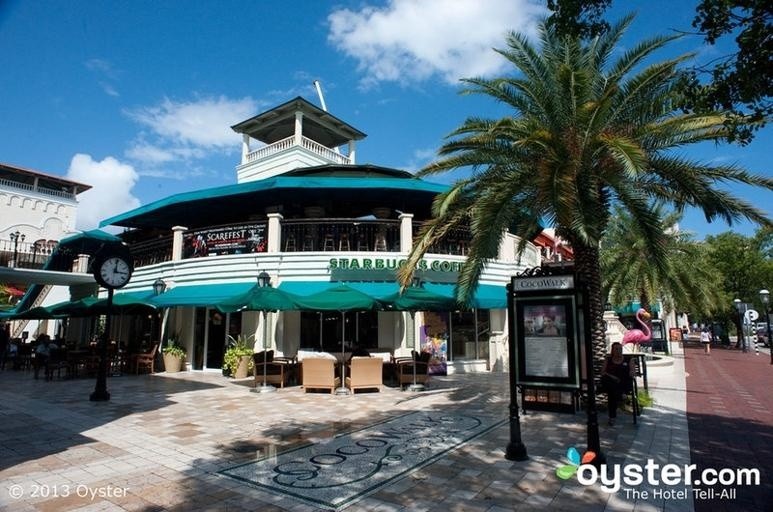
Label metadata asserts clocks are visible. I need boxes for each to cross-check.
[94,253,133,290]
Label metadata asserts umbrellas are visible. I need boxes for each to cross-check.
[216,283,305,386]
[93,291,157,349]
[51,295,107,344]
[294,282,384,387]
[9,306,54,338]
[377,283,460,384]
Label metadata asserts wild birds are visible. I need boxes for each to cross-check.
[621,307,652,353]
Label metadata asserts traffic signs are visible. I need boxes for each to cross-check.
[9,230,26,267]
[759,290,773,364]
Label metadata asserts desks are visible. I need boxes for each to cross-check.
[284,233,387,251]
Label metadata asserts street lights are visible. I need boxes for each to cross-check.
[734,298,747,353]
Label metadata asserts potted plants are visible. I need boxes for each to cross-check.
[221,331,258,379]
[162,327,188,373]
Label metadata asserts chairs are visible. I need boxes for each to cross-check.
[250,349,433,397]
[2,338,158,382]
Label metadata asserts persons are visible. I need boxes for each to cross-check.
[682,326,688,348]
[535,313,560,337]
[0,320,10,370]
[36,334,44,342]
[182,232,207,258]
[94,333,109,355]
[90,332,98,347]
[412,351,421,361]
[139,338,151,354]
[36,336,60,380]
[700,326,713,355]
[524,319,534,335]
[601,342,631,426]
[347,340,371,364]
[6,340,23,371]
